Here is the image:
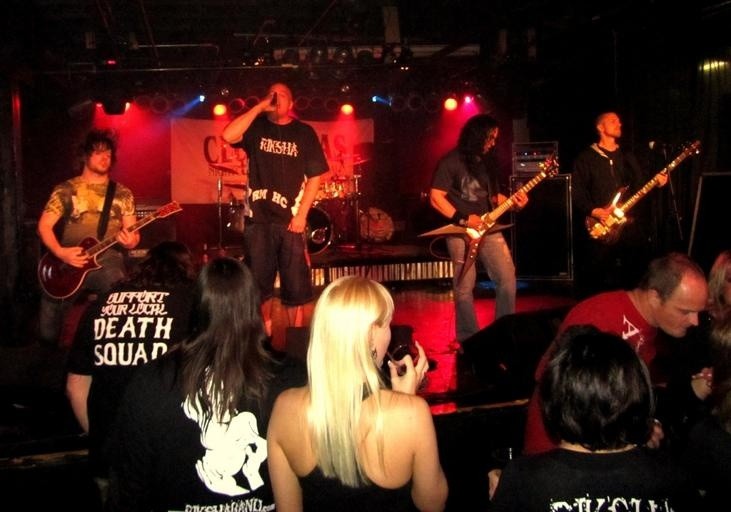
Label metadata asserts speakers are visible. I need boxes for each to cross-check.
[507,172,577,285]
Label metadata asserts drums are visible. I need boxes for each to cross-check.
[304,208,335,257]
[312,195,356,241]
[345,176,365,196]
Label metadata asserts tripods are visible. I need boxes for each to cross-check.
[205,179,241,253]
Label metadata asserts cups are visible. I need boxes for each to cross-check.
[392,345,429,392]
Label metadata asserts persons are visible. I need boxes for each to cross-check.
[35,130,143,362]
[67,238,730,512]
[218,78,332,340]
[573,109,671,302]
[429,113,531,349]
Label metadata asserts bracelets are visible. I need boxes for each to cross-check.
[452,209,469,228]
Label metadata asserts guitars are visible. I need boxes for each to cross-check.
[37,200,183,300]
[417,153,560,287]
[585,139,704,245]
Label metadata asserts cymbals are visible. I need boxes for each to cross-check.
[223,183,248,189]
[209,164,239,174]
[353,158,371,165]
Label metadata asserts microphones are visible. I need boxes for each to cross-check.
[271,93,278,105]
[648,141,675,152]
